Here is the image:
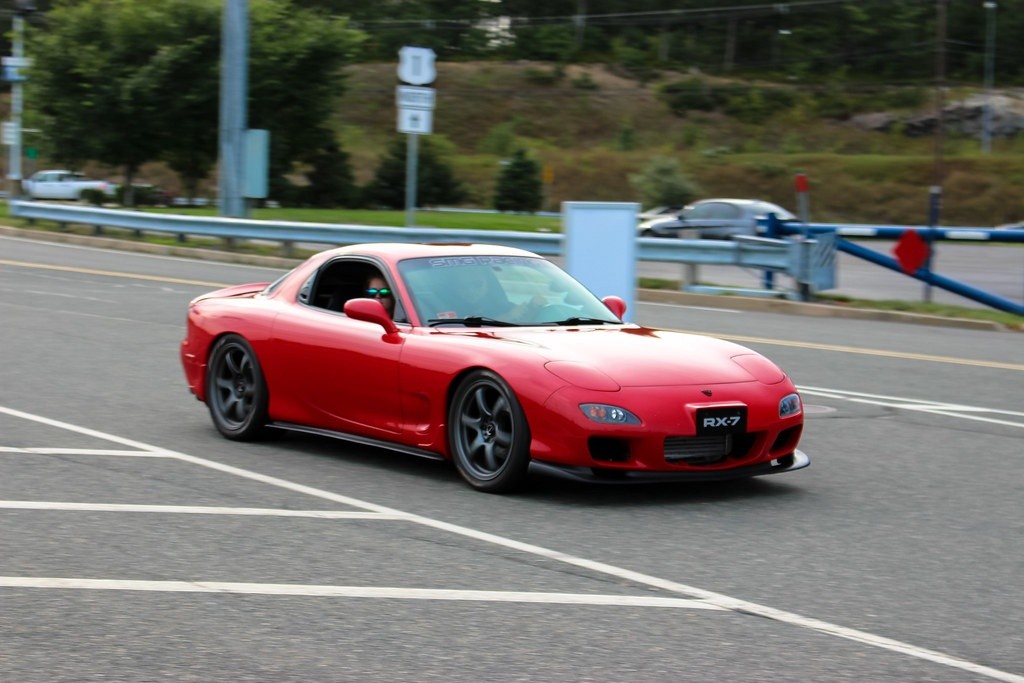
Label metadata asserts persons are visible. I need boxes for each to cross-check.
[367,271,395,318]
[455,271,549,324]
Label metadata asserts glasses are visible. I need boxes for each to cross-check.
[364,288,392,299]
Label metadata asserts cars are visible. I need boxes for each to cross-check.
[20,169,108,201]
[637,197,800,240]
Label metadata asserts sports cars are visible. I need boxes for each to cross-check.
[179,242,813,493]
